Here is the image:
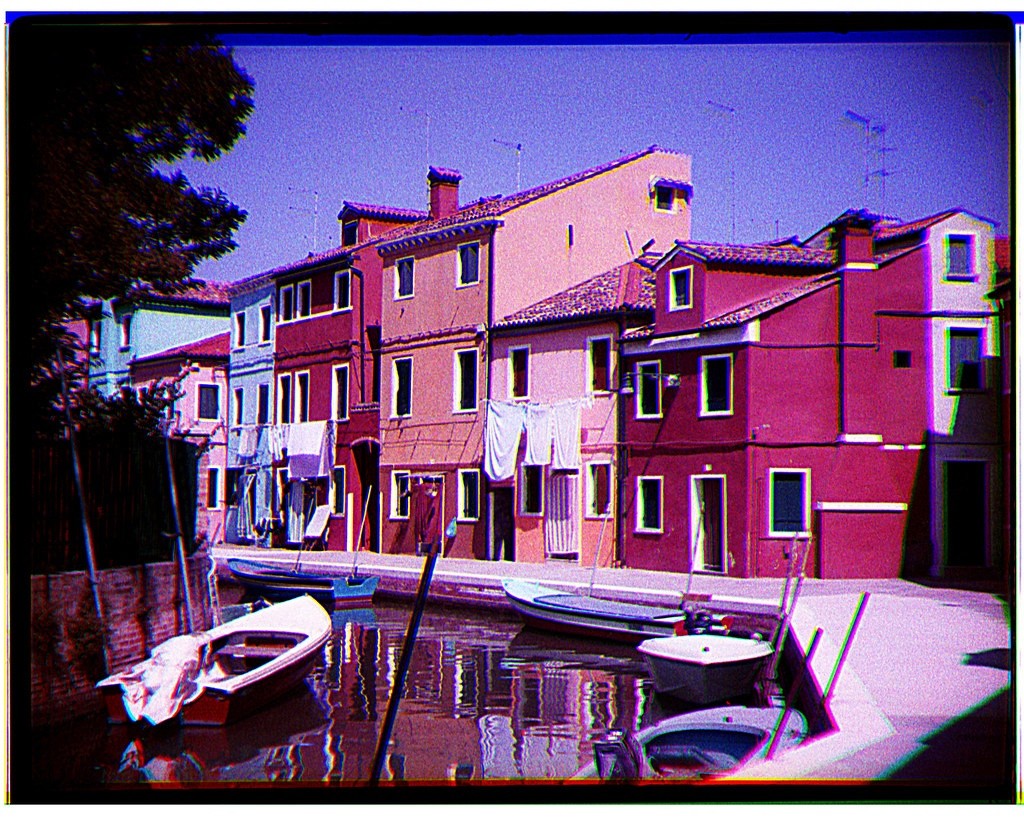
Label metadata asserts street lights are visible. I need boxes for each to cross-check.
[399,106,431,211]
[846,108,872,204]
[288,185,319,252]
[493,139,521,192]
[708,101,737,244]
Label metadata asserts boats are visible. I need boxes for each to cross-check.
[637,616,773,705]
[226,557,380,603]
[564,702,807,785]
[501,578,734,645]
[103,594,338,731]
